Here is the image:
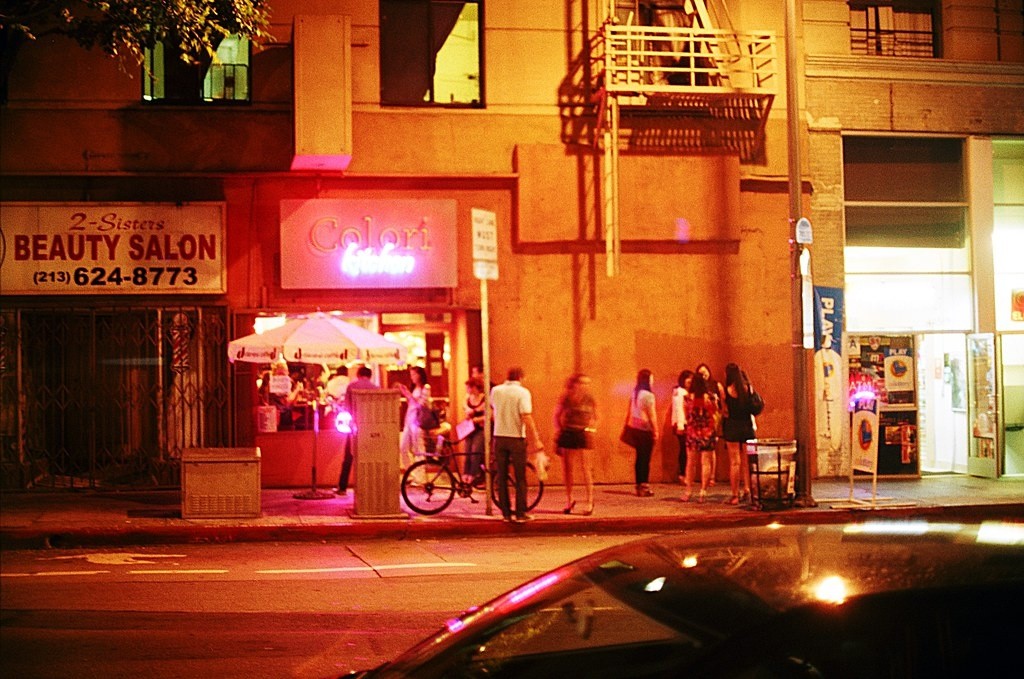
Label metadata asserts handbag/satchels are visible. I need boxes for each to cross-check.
[416,382,440,430]
[746,383,764,415]
[535,449,549,481]
[620,425,646,448]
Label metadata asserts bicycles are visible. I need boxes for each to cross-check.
[401,438,543,515]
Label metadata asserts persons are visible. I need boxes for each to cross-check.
[489,366,544,523]
[620,368,659,497]
[552,374,598,517]
[392,365,431,486]
[332,367,377,496]
[258,364,351,430]
[671,363,765,505]
[461,364,494,493]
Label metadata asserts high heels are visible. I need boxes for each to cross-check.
[563,500,576,514]
[583,502,595,515]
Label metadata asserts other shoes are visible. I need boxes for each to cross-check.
[471,474,485,487]
[503,516,511,523]
[709,478,715,487]
[677,475,694,486]
[724,496,739,505]
[695,490,706,503]
[635,483,654,497]
[515,513,534,523]
[680,490,691,502]
[332,487,346,496]
[739,491,750,503]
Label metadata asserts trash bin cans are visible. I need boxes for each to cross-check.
[745,438,798,512]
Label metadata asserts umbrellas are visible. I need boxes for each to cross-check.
[227,310,408,499]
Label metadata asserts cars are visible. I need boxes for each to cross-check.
[339,518,1024,679]
